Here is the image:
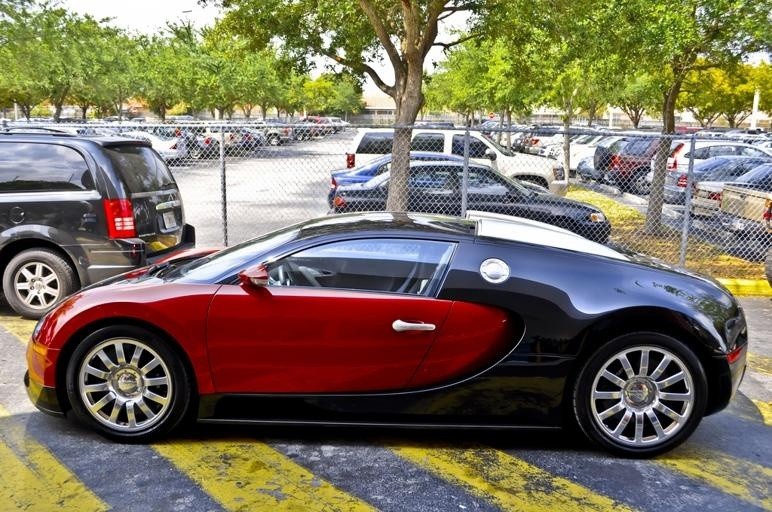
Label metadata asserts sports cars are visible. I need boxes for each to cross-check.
[23,207,750,460]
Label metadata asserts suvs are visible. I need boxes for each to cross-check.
[0,132,197,318]
